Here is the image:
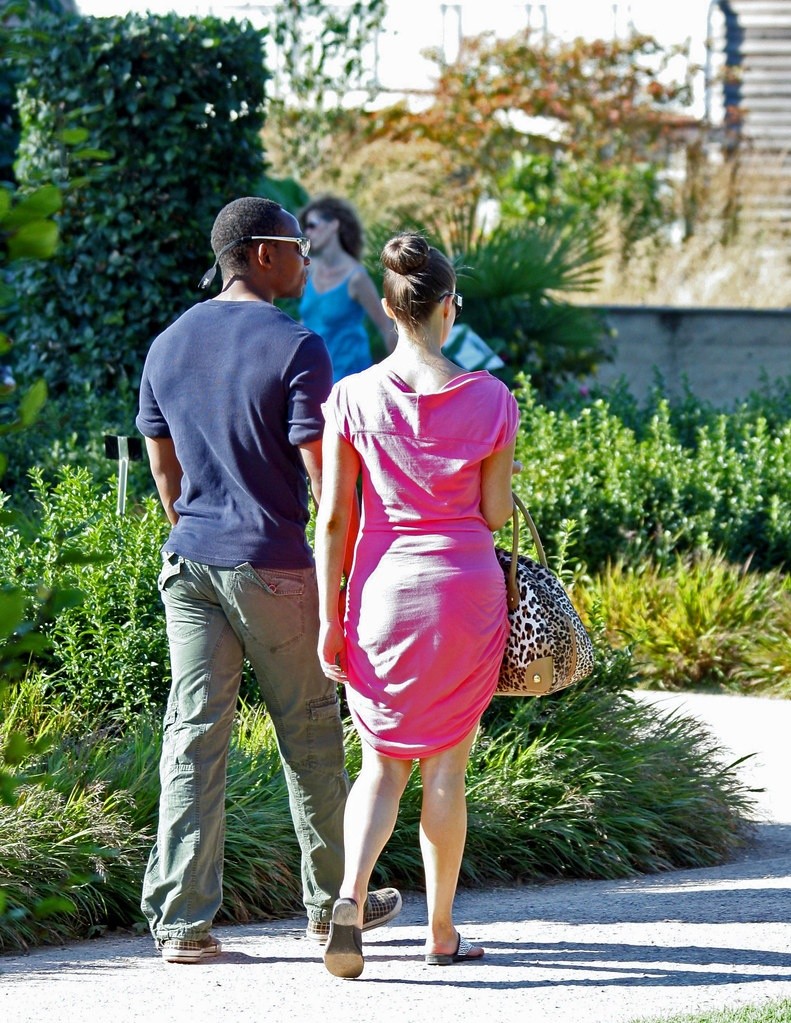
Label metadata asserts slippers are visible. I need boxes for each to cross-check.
[426,932,485,964]
[323,898,365,978]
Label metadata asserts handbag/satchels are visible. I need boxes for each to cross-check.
[494,492,594,696]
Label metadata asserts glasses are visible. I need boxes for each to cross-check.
[438,291,463,318]
[241,234,311,258]
[302,218,330,231]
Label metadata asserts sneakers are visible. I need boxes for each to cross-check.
[306,887,403,940]
[162,931,221,963]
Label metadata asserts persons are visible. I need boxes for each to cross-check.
[313,235,520,977]
[135,197,402,962]
[295,195,398,518]
